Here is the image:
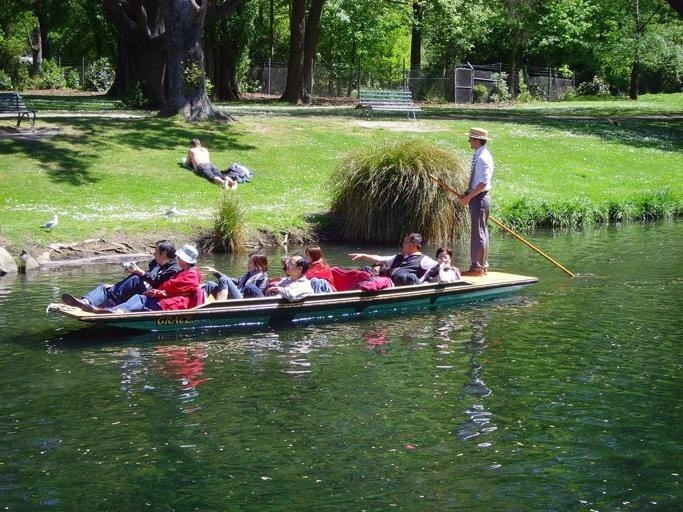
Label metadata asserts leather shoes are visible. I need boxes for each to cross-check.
[461,268,485,276]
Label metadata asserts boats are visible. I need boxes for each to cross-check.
[42,268,541,335]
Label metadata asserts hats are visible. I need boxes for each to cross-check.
[407,233,422,244]
[465,128,492,140]
[175,244,198,264]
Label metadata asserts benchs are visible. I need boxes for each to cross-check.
[354,91,422,120]
[0,92,38,133]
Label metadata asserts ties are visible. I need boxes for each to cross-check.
[469,154,475,181]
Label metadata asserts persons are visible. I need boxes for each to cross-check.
[396,246,461,286]
[62,240,182,310]
[104,244,199,313]
[181,139,238,191]
[198,253,268,299]
[348,233,439,283]
[456,127,494,276]
[241,254,314,302]
[282,245,335,287]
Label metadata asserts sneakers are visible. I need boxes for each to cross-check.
[62,293,85,305]
[82,305,109,314]
